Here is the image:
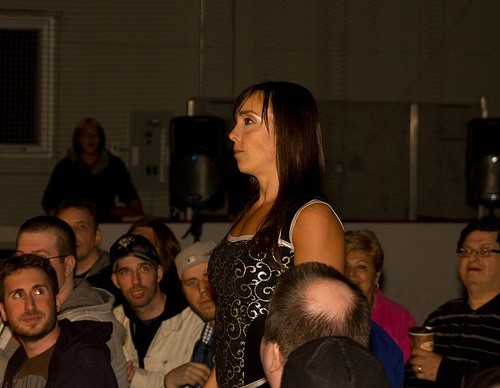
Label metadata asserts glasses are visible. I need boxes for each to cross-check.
[457,246,500,257]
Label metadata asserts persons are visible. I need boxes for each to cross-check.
[40,117,146,224]
[202,80,348,388]
[0,210,500,388]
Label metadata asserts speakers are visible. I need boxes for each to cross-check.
[167,115,227,209]
[467,118,500,207]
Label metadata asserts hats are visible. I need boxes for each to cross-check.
[108,234,160,273]
[278,335,390,387]
[175,240,218,281]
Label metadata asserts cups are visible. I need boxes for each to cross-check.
[408,326,434,365]
